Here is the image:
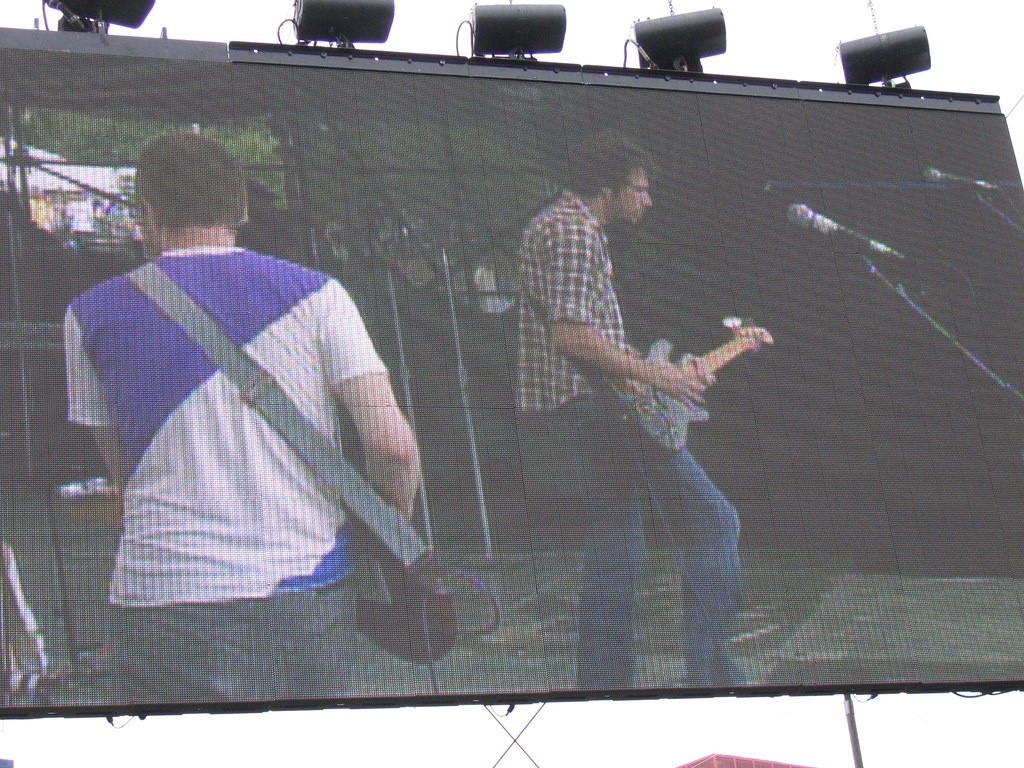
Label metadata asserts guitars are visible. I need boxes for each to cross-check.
[346,520,460,669]
[591,314,776,454]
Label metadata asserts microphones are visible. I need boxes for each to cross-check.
[786,203,904,260]
[922,166,998,192]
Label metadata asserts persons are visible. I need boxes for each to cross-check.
[514,126,752,688]
[64,134,422,706]
